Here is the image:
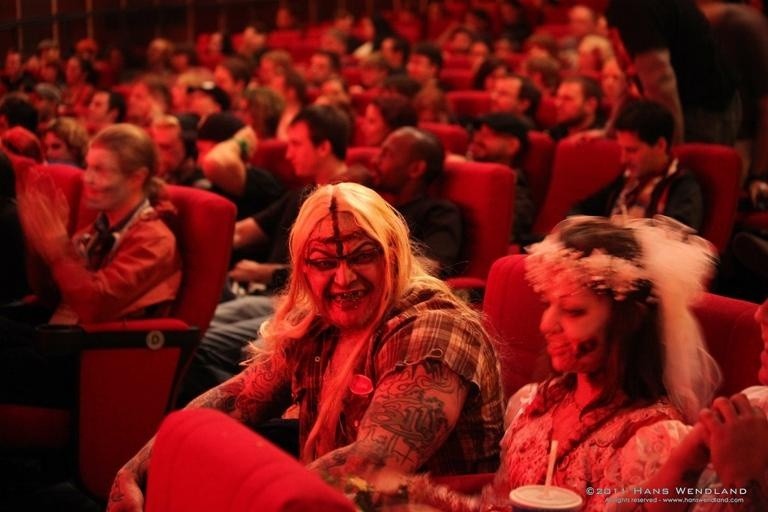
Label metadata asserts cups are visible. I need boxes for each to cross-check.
[509,483,585,512]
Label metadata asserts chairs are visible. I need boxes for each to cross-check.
[0,15,768,512]
